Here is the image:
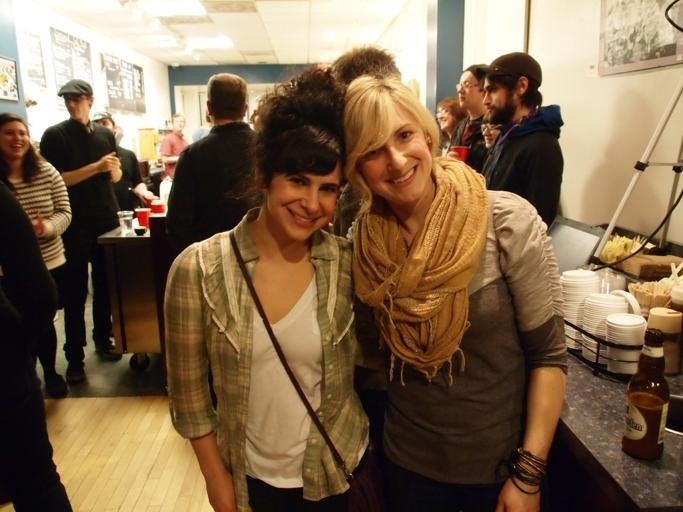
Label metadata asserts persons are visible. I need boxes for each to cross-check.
[156,114,188,181]
[435,95,469,159]
[448,63,493,174]
[93,112,147,210]
[1,112,72,399]
[480,111,502,150]
[164,72,264,263]
[476,52,564,232]
[331,46,402,234]
[163,69,373,512]
[40,79,123,389]
[342,72,568,512]
[0,180,74,512]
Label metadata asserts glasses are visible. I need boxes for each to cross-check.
[435,108,449,117]
[455,82,480,88]
[481,123,504,131]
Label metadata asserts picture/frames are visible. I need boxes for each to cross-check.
[0,56,20,103]
[597,0,683,77]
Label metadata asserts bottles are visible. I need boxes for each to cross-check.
[624,329,671,460]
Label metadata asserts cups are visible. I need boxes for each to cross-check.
[146,198,152,207]
[151,203,165,214]
[117,210,134,233]
[135,208,152,229]
[451,145,468,165]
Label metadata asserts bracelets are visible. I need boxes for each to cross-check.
[506,447,548,496]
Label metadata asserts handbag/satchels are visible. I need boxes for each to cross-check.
[230,227,385,512]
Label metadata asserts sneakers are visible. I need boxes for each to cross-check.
[44,374,67,396]
[66,363,83,386]
[95,342,122,361]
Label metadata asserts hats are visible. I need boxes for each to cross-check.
[91,111,116,124]
[58,79,93,97]
[476,52,543,89]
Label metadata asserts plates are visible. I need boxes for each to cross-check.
[554,270,600,349]
[606,313,647,375]
[582,290,641,365]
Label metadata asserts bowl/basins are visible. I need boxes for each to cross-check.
[135,228,147,236]
